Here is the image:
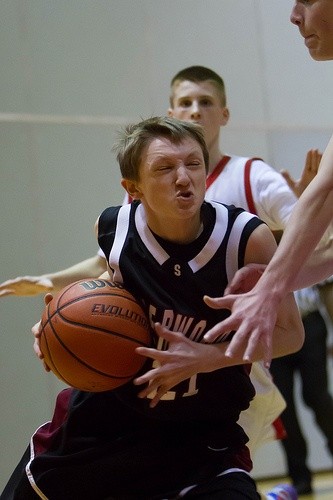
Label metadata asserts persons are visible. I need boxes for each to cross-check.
[265,279,333,500]
[165,64,301,451]
[1,114,307,500]
[201,0,333,370]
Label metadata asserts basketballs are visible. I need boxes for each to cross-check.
[40,279,151,392]
